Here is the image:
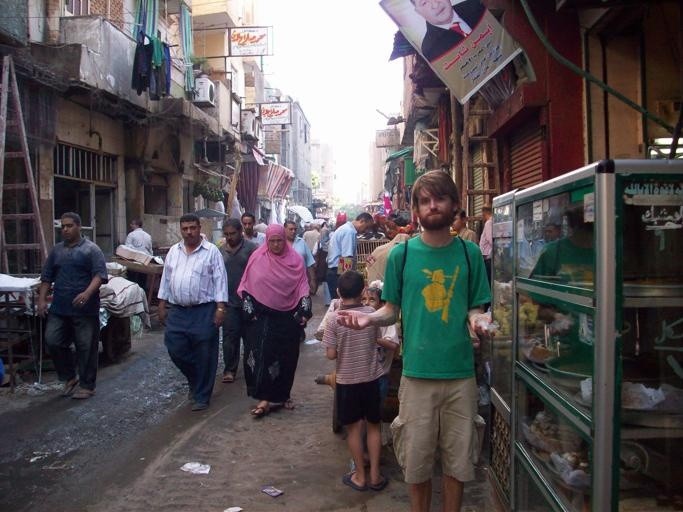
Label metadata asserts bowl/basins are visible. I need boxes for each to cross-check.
[543,354,594,391]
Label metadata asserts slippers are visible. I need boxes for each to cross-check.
[283,399,295,410]
[370,473,387,491]
[251,406,272,417]
[222,373,234,382]
[61,379,80,394]
[72,387,97,399]
[344,471,367,491]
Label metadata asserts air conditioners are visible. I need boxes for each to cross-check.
[241,111,256,138]
[256,118,260,140]
[193,78,216,107]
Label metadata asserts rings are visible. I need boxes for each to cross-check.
[80,301,83,303]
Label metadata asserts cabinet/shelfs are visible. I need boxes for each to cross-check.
[490,159,683,512]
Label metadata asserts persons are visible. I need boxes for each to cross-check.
[253,218,268,233]
[39,212,108,399]
[337,170,496,511]
[125,219,153,293]
[452,207,477,245]
[322,272,389,490]
[324,213,374,299]
[530,205,596,457]
[409,1,485,63]
[237,224,312,415]
[220,219,258,382]
[240,213,266,245]
[478,204,492,288]
[299,220,331,254]
[350,281,400,470]
[284,221,318,294]
[157,213,230,412]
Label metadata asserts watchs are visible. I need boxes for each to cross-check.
[217,308,222,311]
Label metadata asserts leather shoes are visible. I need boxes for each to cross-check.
[188,389,196,400]
[192,400,208,410]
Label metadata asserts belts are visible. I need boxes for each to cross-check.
[176,303,203,309]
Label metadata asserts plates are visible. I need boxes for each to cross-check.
[572,390,592,407]
[621,408,682,428]
[531,360,550,372]
[529,274,564,279]
[567,281,594,286]
[621,283,682,297]
[549,440,649,495]
